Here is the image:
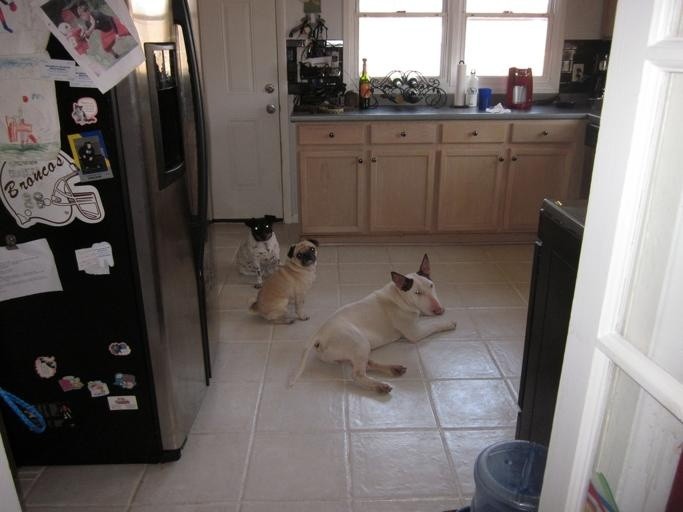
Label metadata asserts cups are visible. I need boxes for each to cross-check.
[478,87,492,111]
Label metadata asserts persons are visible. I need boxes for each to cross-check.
[58,15,108,77]
[77,141,95,175]
[74,4,131,64]
[0,0,17,35]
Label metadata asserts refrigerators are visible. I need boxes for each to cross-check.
[0,0,222,468]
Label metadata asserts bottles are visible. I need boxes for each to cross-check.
[465,68,480,108]
[358,58,371,110]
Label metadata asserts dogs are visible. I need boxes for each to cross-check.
[234,215,282,289]
[285,251,457,396]
[248,237,321,326]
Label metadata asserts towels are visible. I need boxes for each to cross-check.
[485,102,512,114]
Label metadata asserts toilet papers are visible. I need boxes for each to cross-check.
[454,65,466,106]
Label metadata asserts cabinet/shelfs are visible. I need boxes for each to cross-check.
[300,124,439,235]
[513,194,587,443]
[438,120,588,235]
